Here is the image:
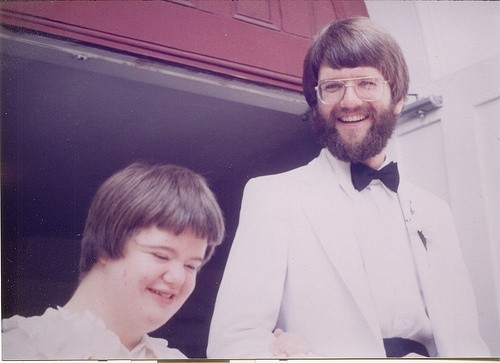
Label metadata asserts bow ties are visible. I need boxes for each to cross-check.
[350,159,400,193]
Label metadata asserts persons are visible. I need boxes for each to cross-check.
[3,157,225,363]
[206,14,489,359]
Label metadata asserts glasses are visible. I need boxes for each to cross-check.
[314,74,391,106]
